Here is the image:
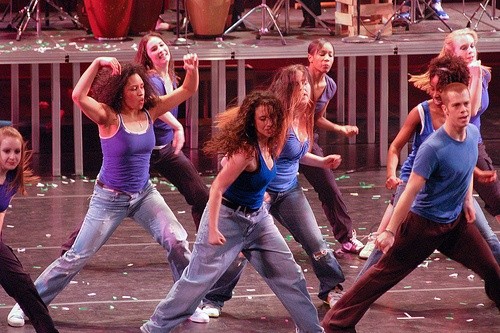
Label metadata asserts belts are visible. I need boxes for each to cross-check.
[97,180,123,193]
[222,199,259,213]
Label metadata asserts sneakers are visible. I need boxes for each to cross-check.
[7,303,26,327]
[359,232,380,259]
[342,229,364,253]
[324,286,345,308]
[428,0,449,19]
[202,304,220,317]
[189,307,209,323]
[398,11,409,18]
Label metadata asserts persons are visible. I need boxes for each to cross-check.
[323,83,499,332]
[355,56,500,280]
[306,39,363,251]
[359,29,499,260]
[60,33,210,256]
[1,127,57,332]
[140,92,325,333]
[202,65,344,315]
[8,54,210,327]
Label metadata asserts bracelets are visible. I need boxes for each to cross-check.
[384,229,396,238]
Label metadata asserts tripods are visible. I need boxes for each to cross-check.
[216,0,335,45]
[466,0,500,31]
[0,0,93,41]
[374,0,452,41]
